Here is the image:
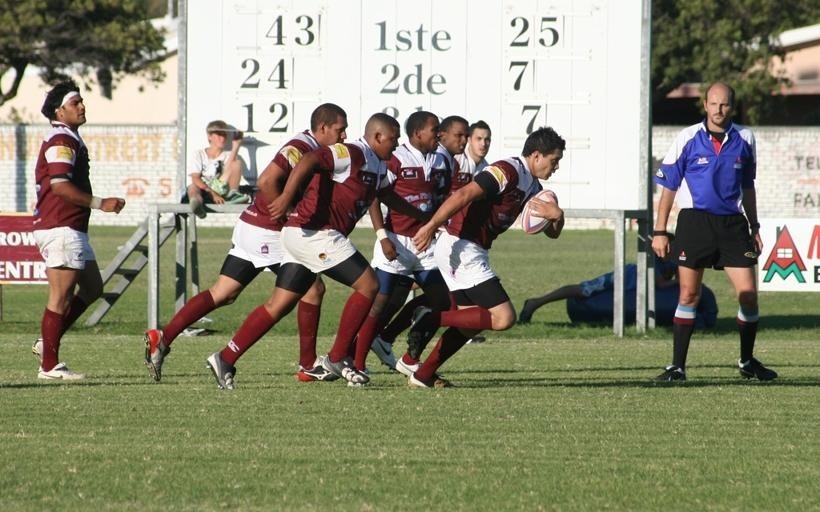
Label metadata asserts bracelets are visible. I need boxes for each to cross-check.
[89,195,103,210]
[650,230,667,237]
[750,223,761,231]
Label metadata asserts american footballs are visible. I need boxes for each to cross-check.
[523,190,557,236]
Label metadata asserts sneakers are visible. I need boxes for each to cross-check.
[407,304,435,359]
[145,329,170,381]
[653,365,686,381]
[738,360,777,379]
[396,356,420,376]
[411,371,449,388]
[322,354,369,383]
[371,334,396,368]
[33,339,44,363]
[298,357,339,381]
[466,335,485,344]
[222,193,248,203]
[207,352,236,390]
[190,198,206,218]
[38,362,84,380]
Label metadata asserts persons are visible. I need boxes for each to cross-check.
[517,230,682,326]
[646,78,778,383]
[209,110,567,392]
[33,78,127,383]
[144,101,348,382]
[185,120,249,219]
